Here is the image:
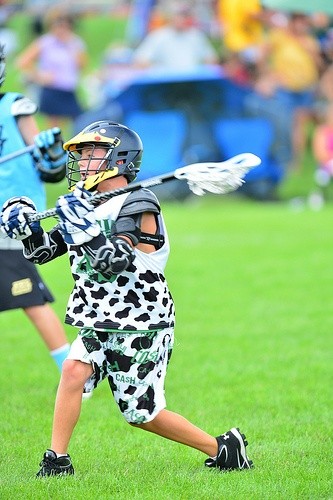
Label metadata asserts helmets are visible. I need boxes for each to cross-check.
[63,120,143,193]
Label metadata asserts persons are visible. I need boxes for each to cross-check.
[1,0,333,193]
[0,42,95,400]
[0,121,252,474]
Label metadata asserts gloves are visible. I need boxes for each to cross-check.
[0,196,43,242]
[33,127,68,184]
[55,194,99,245]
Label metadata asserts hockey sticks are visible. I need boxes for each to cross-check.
[1,127,62,164]
[28,152,263,224]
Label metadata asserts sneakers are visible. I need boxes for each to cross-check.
[36,449,73,479]
[205,428,255,471]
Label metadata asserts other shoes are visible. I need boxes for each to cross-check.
[81,392,91,398]
